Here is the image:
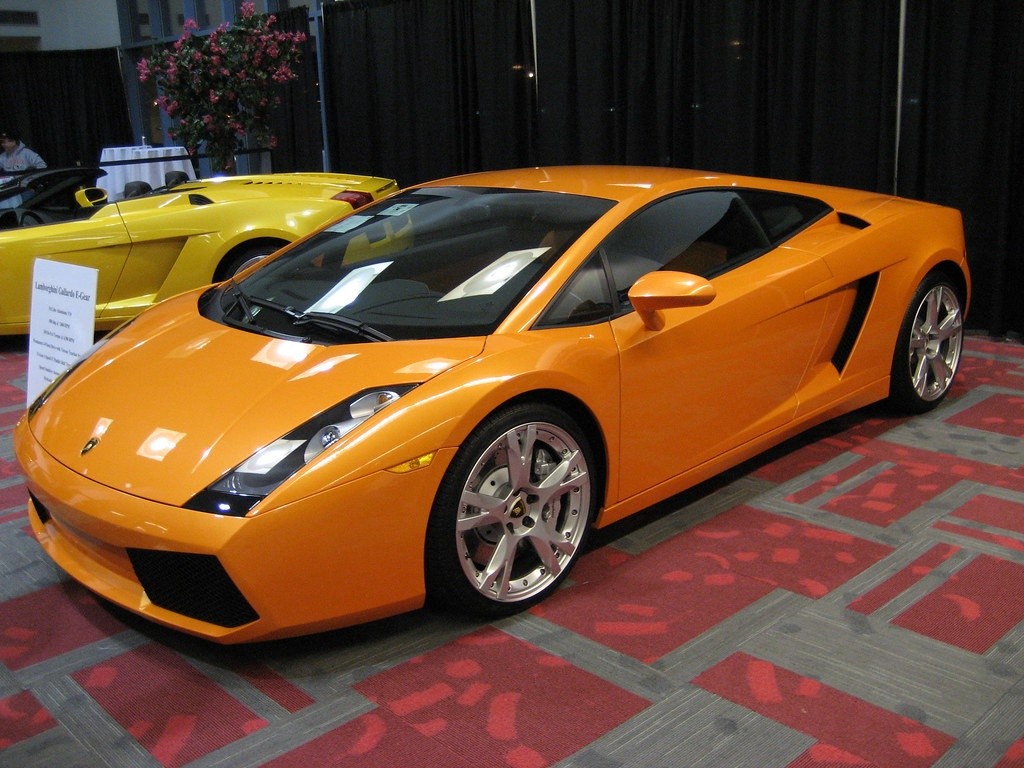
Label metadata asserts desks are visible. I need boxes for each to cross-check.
[96,145,155,202]
[131,147,197,191]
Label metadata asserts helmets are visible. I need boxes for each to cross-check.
[0,131,16,140]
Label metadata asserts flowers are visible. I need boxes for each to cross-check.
[137,1,308,174]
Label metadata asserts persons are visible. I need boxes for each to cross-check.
[0,131,48,223]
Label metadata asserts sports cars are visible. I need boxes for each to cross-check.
[12,164,971,643]
[0,165,414,335]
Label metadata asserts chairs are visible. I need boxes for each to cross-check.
[164,171,189,189]
[123,181,150,199]
